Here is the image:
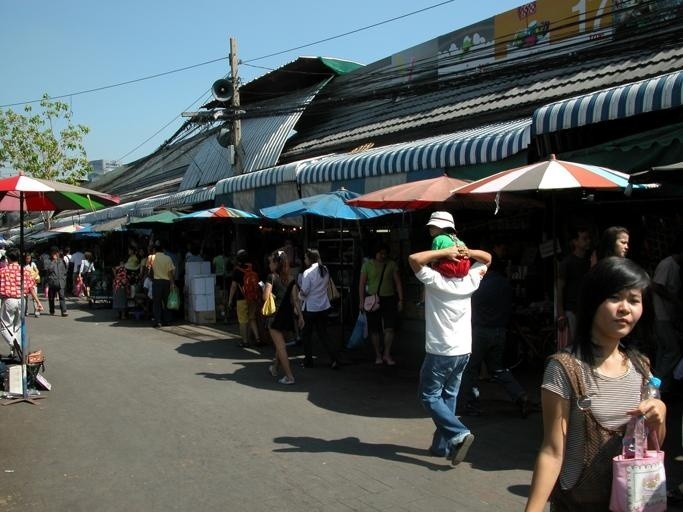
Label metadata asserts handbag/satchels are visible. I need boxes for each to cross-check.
[260,292,276,316]
[364,294,380,312]
[327,277,340,300]
[346,309,368,350]
[609,416,668,512]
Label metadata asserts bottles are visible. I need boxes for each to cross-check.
[639,377,661,406]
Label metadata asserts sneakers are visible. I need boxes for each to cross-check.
[456,400,480,415]
[268,365,295,384]
[35,311,40,316]
[516,392,529,417]
[452,433,475,465]
[62,311,67,316]
[376,356,395,365]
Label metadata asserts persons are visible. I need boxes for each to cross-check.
[426,211,472,278]
[484,236,508,258]
[0,238,203,358]
[212,239,338,385]
[358,242,404,367]
[525,256,667,512]
[556,225,592,349]
[461,250,529,419]
[651,243,683,395]
[596,225,631,263]
[407,246,494,466]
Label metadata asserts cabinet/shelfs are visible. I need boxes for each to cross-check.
[317,238,356,317]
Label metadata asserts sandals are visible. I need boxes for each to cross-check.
[238,341,249,347]
[255,338,262,345]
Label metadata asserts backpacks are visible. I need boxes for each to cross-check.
[234,263,259,301]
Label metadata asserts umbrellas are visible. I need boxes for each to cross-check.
[342,172,519,212]
[453,152,663,350]
[0,171,122,407]
[28,204,261,324]
[258,184,407,357]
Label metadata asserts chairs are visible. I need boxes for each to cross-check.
[13,338,44,390]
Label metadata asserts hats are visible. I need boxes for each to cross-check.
[426,211,456,232]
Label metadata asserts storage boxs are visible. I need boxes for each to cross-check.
[184,261,216,324]
